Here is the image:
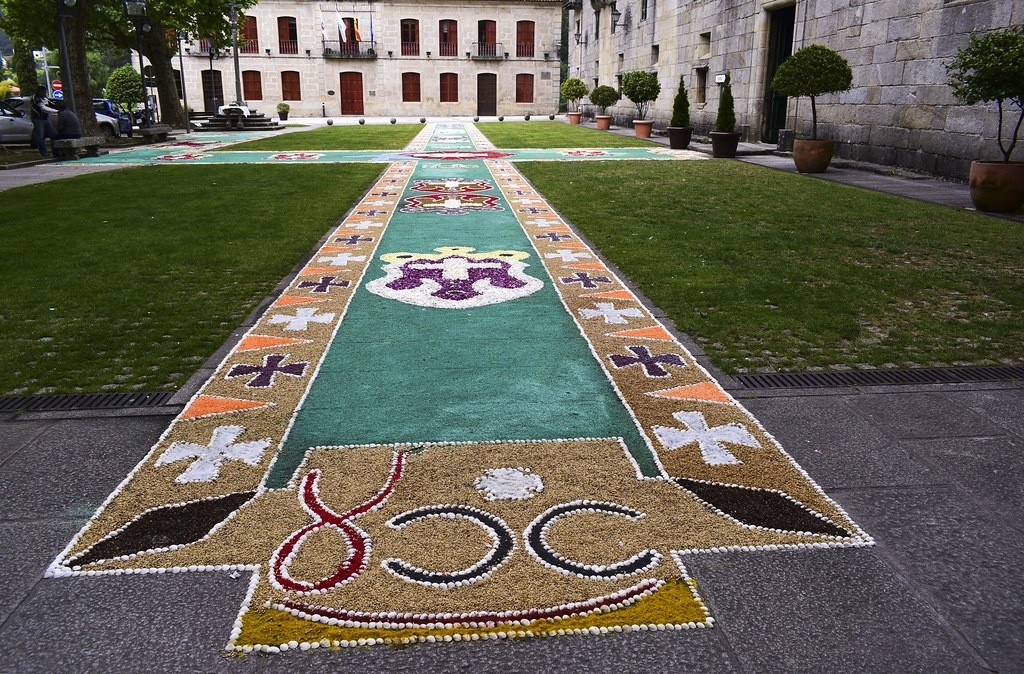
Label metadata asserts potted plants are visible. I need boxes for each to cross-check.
[276,103,290,121]
[588,85,619,130]
[769,42,854,174]
[560,77,587,124]
[938,26,1024,215]
[620,70,661,138]
[708,68,742,158]
[665,74,694,149]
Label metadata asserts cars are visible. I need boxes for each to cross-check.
[0,99,48,150]
[13,96,120,143]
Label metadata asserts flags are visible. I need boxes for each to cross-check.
[337,12,347,43]
[353,16,361,41]
[370,15,375,36]
[320,10,328,39]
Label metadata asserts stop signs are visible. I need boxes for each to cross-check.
[52,80,64,90]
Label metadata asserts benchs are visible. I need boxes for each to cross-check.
[52,135,104,159]
[139,123,167,127]
[136,126,172,143]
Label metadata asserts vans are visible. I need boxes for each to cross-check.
[92,98,134,139]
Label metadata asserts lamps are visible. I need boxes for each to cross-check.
[544,52,548,59]
[305,49,309,55]
[465,51,470,57]
[387,50,392,57]
[265,49,270,55]
[185,47,189,53]
[426,51,430,56]
[609,8,628,26]
[504,52,508,58]
[225,48,230,54]
[574,31,586,44]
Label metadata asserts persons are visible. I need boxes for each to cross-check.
[30,86,59,156]
[52,100,81,157]
